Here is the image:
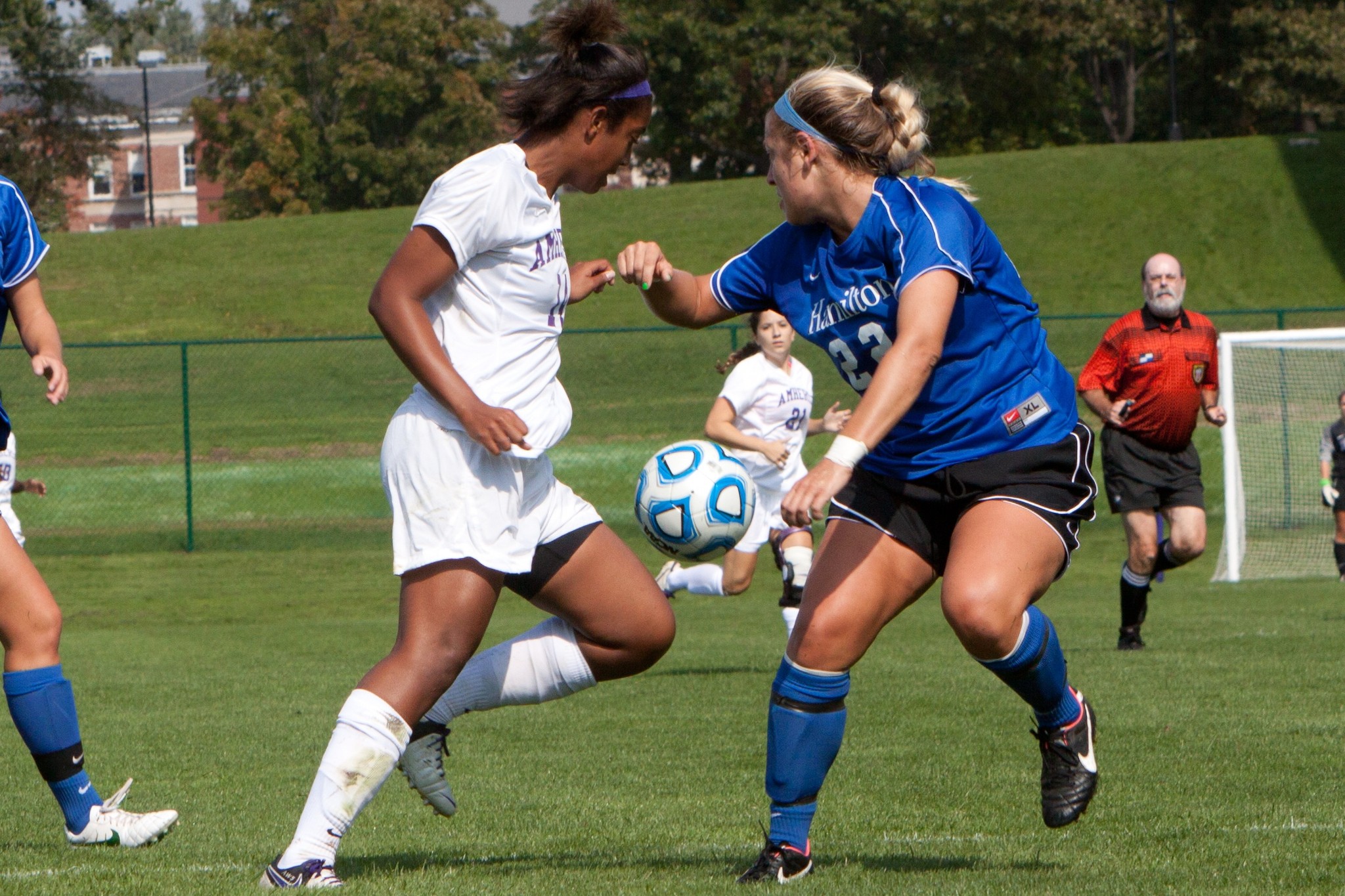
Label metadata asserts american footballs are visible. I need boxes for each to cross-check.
[634,440,758,562]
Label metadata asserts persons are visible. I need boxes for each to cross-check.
[0,176,178,850]
[617,52,1101,883]
[1320,391,1345,583]
[654,310,854,640]
[1075,252,1226,648]
[257,0,675,888]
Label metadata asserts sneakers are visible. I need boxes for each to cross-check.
[399,720,459,817]
[258,854,343,887]
[63,777,179,848]
[1028,684,1098,828]
[654,560,681,600]
[738,820,814,884]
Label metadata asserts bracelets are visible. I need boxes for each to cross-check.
[1205,404,1216,409]
[1321,477,1331,486]
[823,434,868,469]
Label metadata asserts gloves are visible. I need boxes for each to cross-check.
[1318,478,1339,506]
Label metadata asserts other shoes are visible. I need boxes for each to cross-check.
[1117,625,1143,649]
